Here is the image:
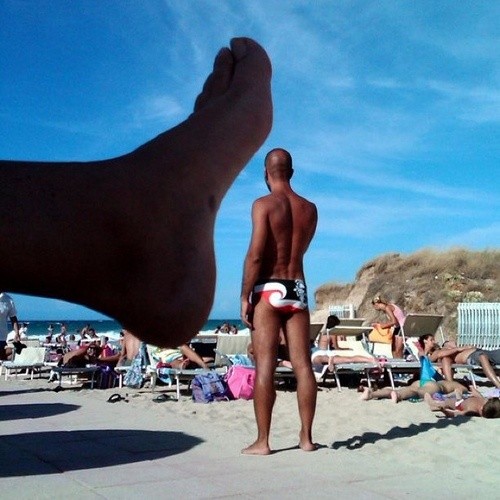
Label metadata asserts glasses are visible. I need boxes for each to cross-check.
[88,347,96,350]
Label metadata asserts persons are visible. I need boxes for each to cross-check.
[45,323,208,388]
[419,334,500,387]
[371,296,404,351]
[0,292,20,361]
[0,38,272,349]
[240,149,318,454]
[425,387,500,419]
[8,323,27,341]
[360,380,468,403]
[247,315,372,369]
[215,323,237,334]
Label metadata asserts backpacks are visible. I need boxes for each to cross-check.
[189,370,229,403]
[222,365,255,400]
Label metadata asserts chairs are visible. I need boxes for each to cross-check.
[0,301,499,398]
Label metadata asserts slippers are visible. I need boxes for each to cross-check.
[151,394,178,402]
[105,394,125,402]
